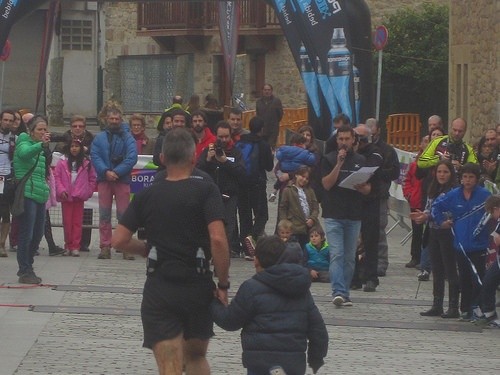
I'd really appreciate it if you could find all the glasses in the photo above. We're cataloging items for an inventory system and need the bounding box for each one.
[71,125,85,129]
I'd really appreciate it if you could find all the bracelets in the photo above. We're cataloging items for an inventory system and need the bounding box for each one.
[218,281,230,289]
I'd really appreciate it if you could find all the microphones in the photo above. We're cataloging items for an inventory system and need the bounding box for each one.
[341,144,347,161]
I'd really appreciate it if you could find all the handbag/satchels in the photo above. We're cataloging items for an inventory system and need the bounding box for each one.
[3,179,28,217]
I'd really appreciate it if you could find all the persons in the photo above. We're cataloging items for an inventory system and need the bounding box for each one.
[409,160,460,318]
[209,235,329,375]
[475,196,500,328]
[319,127,371,306]
[53,139,97,256]
[268,114,400,292]
[256,84,283,150]
[110,128,230,375]
[153,94,274,261]
[0,110,69,257]
[431,162,493,322]
[52,115,94,153]
[13,119,52,284]
[400,115,500,281]
[89,109,138,260]
[129,115,149,154]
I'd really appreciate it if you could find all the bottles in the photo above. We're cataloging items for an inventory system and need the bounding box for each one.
[316,55,325,75]
[299,41,315,73]
[326,26,350,77]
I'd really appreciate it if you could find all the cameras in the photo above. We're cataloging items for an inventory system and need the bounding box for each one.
[213,137,228,154]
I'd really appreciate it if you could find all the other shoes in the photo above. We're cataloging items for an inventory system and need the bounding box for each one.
[16,271,35,277]
[377,268,387,277]
[18,274,41,284]
[362,280,375,292]
[123,252,135,259]
[63,249,71,256]
[475,311,498,327]
[342,297,353,306]
[49,246,67,256]
[441,308,461,318]
[457,311,473,321]
[348,280,362,290]
[71,249,80,257]
[10,248,17,252]
[245,235,256,256]
[98,247,111,259]
[417,270,429,280]
[332,294,344,306]
[405,259,420,267]
[231,250,240,258]
[269,193,276,202]
[419,303,444,316]
[243,251,255,260]
[0,245,7,257]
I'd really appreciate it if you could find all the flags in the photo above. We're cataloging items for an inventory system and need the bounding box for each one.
[267,0,376,132]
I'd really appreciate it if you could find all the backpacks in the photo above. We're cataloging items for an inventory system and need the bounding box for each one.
[223,137,265,178]
